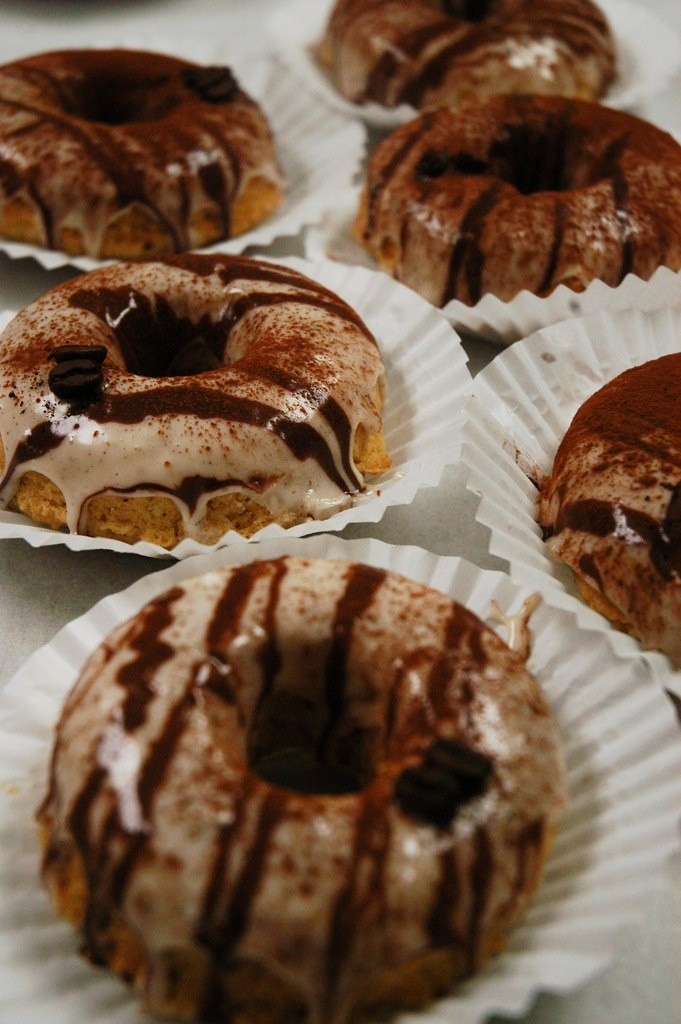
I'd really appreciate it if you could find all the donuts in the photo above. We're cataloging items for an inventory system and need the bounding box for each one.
[1,0,681,1024]
